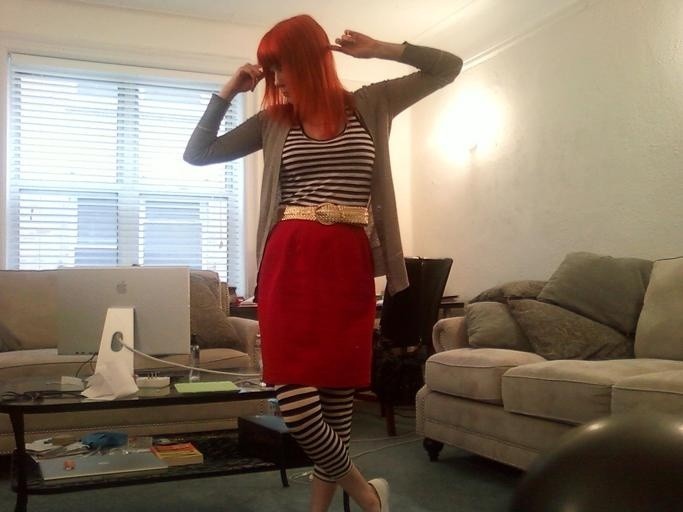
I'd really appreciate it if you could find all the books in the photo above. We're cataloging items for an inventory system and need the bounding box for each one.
[174,381,241,395]
[25,434,91,464]
[240,295,258,308]
[151,442,203,467]
[132,347,252,374]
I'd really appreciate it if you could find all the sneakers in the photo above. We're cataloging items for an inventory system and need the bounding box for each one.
[367,477,390,512]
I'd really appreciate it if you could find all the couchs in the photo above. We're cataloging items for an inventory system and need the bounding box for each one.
[0,264,258,457]
[414,250,680,473]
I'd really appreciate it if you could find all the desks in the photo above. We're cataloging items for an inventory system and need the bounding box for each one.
[226,291,465,403]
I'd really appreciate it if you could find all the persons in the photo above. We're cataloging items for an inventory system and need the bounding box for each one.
[183,15,462,512]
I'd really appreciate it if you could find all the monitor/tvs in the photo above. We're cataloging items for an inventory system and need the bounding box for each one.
[57,267,191,390]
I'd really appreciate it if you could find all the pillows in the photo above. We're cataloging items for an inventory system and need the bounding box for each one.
[461,300,536,355]
[185,267,242,349]
[536,249,652,336]
[509,299,633,361]
[466,277,548,306]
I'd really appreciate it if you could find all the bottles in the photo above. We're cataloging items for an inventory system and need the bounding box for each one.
[187,333,201,381]
[229,286,238,306]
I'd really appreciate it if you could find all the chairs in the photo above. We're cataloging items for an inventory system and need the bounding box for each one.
[353,258,452,432]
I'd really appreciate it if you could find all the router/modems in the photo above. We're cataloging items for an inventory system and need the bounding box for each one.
[136,376,171,388]
